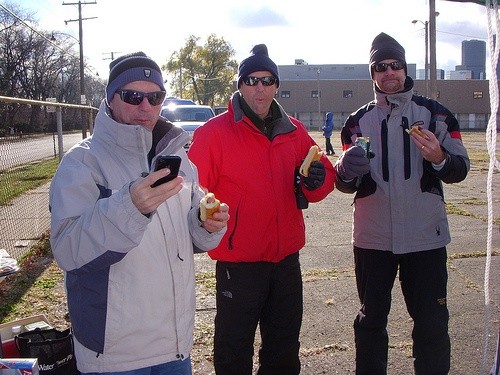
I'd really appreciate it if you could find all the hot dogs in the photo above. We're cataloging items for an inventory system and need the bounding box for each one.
[200,192,220,221]
[405,126,427,139]
[299,145,324,178]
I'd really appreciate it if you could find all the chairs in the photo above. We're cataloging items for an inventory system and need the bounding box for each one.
[166,114,175,121]
[196,113,205,120]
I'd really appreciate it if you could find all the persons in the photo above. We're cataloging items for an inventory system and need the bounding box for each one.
[333,32,470,375]
[188,44,336,375]
[49,52,230,375]
[322,112,335,155]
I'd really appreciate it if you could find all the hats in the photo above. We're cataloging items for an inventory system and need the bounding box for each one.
[238,44,280,87]
[105,51,165,101]
[369,32,407,76]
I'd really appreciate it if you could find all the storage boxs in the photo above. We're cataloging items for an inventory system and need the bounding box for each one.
[0,314,50,360]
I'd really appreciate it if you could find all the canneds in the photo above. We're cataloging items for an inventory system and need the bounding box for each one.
[355,136,371,159]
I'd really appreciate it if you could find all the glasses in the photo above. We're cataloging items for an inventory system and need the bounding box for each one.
[116,88,165,106]
[375,61,405,72]
[243,76,277,86]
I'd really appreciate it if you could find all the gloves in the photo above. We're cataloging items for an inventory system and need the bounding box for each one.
[337,147,375,180]
[301,159,326,189]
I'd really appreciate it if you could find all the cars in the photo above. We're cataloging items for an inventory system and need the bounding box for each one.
[160,105,228,156]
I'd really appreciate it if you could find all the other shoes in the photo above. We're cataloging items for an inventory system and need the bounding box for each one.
[326,151,330,155]
[331,152,335,154]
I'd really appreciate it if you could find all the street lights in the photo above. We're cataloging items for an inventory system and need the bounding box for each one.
[316,68,323,132]
[411,11,441,99]
[50,30,87,140]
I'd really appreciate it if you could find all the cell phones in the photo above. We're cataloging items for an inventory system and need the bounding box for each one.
[153,155,181,187]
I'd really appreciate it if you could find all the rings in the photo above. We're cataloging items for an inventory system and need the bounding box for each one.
[421,146,425,150]
